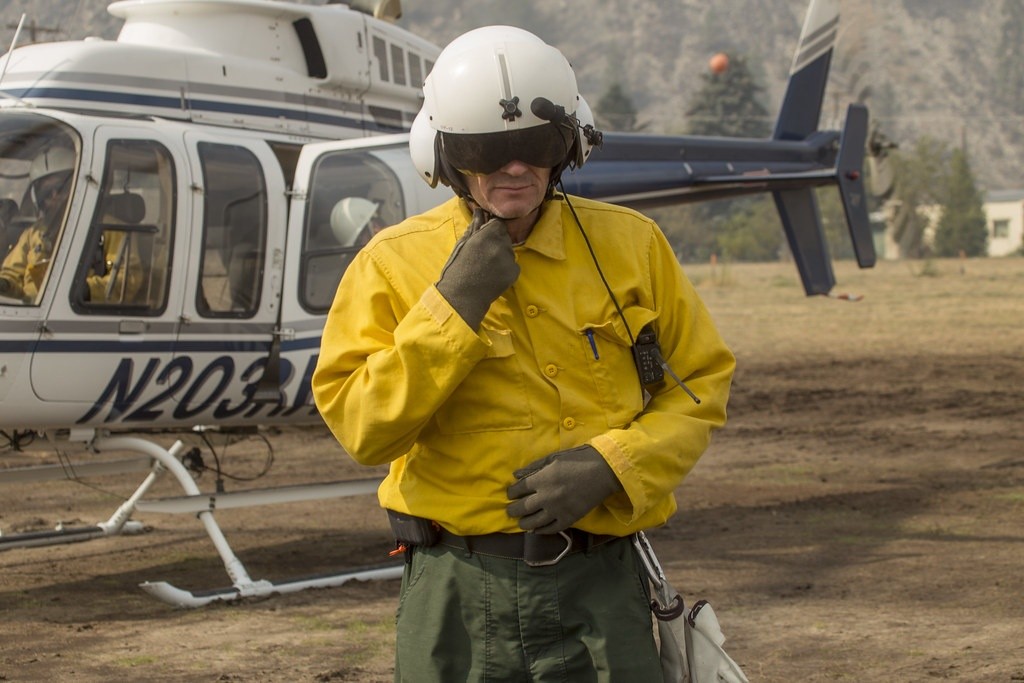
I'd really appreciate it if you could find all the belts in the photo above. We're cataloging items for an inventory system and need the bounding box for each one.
[432,522,616,566]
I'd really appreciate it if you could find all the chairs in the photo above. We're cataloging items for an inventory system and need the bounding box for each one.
[108,191,156,307]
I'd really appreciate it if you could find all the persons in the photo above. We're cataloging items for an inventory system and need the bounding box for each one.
[312,26,737,683]
[0,146,144,302]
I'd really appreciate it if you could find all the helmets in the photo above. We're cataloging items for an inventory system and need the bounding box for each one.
[29,147,76,185]
[329,195,381,257]
[410,24,594,189]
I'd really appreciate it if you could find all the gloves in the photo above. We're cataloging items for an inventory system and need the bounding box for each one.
[433,204,522,333]
[500,444,625,535]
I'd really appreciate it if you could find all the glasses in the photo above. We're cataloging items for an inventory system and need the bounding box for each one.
[438,121,577,174]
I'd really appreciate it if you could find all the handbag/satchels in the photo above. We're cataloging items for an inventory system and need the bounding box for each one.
[648,591,749,683]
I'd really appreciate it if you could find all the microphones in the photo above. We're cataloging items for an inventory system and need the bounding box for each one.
[531,97,604,154]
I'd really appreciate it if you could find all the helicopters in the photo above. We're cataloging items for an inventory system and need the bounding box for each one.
[0,0,876,608]
